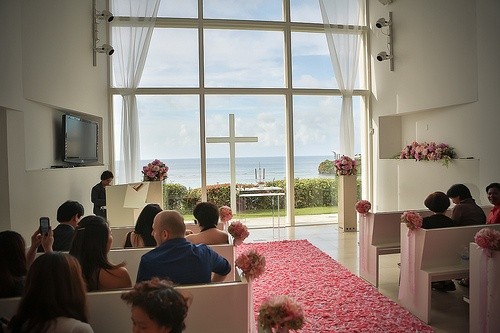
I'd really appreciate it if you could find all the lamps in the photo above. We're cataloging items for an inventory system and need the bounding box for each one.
[377,51,390,62]
[96,9,114,23]
[96,44,115,56]
[376,18,389,29]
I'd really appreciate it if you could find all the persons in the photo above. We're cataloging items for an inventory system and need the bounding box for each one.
[37,200,84,253]
[0,228,54,299]
[458,183,500,288]
[421,192,456,291]
[69,215,132,290]
[121,280,189,333]
[183,202,229,244]
[447,184,486,226]
[136,210,231,284]
[124,204,163,249]
[91,171,114,219]
[7,250,93,333]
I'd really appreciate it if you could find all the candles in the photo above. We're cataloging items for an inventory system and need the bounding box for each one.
[263,168,265,179]
[255,168,257,179]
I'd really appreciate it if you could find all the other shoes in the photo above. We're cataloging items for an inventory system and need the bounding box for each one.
[434,282,456,291]
[458,278,469,287]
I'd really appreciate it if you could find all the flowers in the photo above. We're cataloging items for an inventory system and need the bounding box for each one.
[354,199,372,215]
[400,140,457,169]
[233,247,267,280]
[257,293,304,333]
[333,154,358,176]
[473,227,500,257]
[399,211,426,232]
[228,220,251,245]
[141,159,170,182]
[218,205,232,222]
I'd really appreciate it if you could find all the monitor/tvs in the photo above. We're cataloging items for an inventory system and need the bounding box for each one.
[60,115,99,163]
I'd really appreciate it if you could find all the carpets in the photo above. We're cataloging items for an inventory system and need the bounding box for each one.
[227,239,432,333]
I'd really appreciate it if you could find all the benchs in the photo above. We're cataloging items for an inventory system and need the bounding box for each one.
[356,211,500,333]
[0,221,252,333]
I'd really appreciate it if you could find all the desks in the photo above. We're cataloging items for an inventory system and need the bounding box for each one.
[237,188,286,219]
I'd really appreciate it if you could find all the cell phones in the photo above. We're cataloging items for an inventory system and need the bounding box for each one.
[40,217,50,236]
